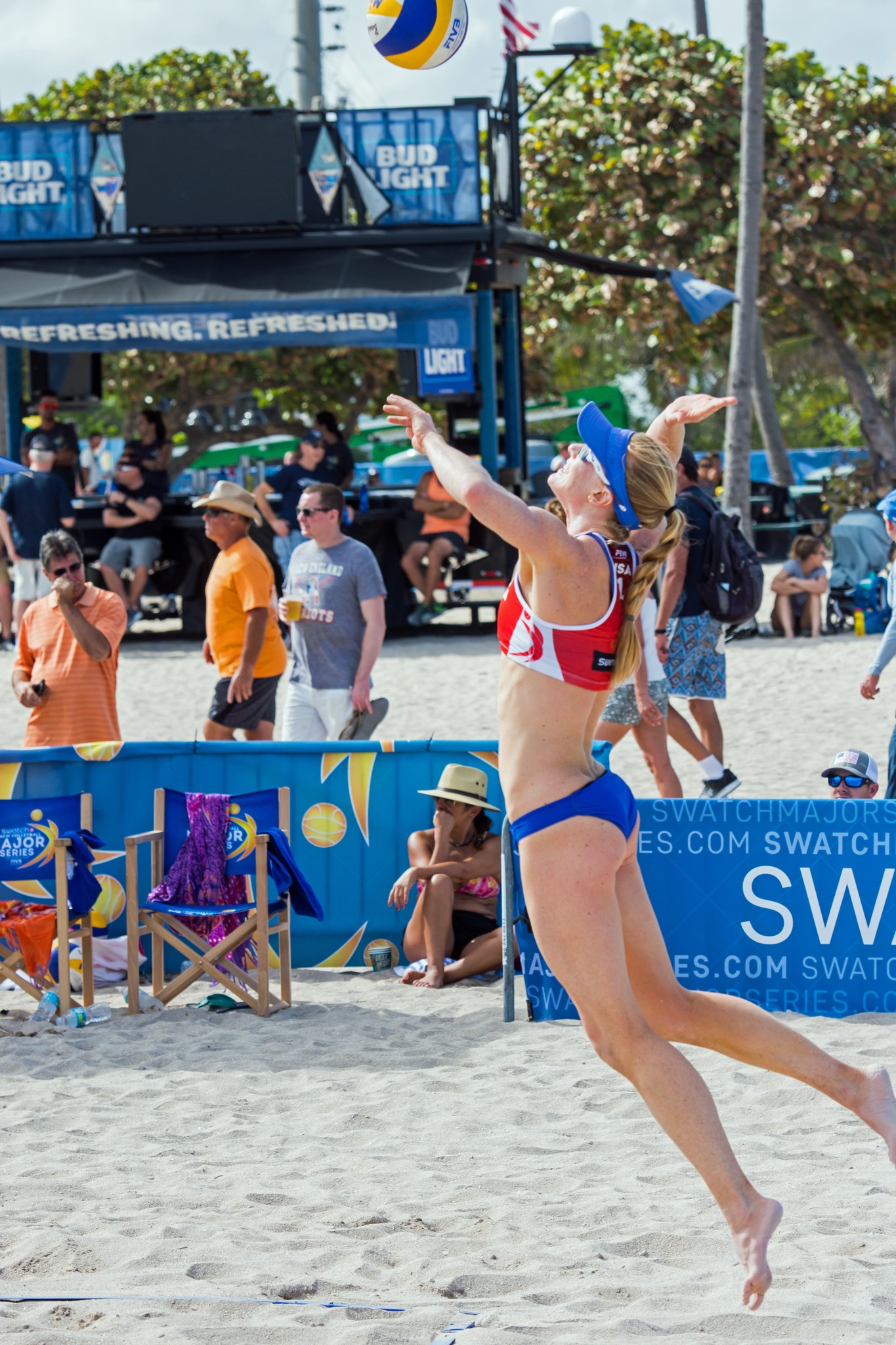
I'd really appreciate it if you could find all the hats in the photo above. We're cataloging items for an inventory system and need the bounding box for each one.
[118,453,142,466]
[417,763,502,813]
[679,446,699,471]
[822,749,878,784]
[316,411,345,440]
[302,429,330,445]
[191,480,264,528]
[577,400,644,531]
[30,434,57,452]
[876,488,896,522]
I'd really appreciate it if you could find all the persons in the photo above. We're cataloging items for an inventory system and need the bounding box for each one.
[861,489,896,799]
[0,536,13,645]
[19,387,77,500]
[98,461,162,630]
[278,483,387,742]
[80,428,112,496]
[770,535,830,638]
[0,435,76,645]
[387,763,519,990]
[821,748,878,799]
[382,393,896,1312]
[592,516,687,798]
[252,431,354,582]
[192,481,287,742]
[116,407,173,496]
[314,409,355,491]
[401,470,470,626]
[653,445,742,799]
[11,529,128,749]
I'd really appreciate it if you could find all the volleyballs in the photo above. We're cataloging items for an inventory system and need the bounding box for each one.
[42,941,83,993]
[365,0,468,70]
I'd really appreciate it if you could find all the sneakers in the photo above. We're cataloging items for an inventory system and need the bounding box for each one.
[699,768,742,799]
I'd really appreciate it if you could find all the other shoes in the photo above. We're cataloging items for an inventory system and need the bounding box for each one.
[126,608,143,629]
[0,633,15,651]
[408,602,445,625]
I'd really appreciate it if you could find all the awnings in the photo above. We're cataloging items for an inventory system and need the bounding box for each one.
[0,457,34,476]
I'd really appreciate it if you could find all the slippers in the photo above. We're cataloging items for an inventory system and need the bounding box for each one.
[186,993,252,1013]
[339,698,388,740]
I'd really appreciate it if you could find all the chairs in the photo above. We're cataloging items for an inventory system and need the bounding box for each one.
[125,787,291,1017]
[0,792,94,1015]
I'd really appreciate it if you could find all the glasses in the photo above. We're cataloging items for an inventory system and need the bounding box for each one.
[432,795,458,807]
[701,464,712,468]
[51,560,82,577]
[205,506,234,519]
[41,402,60,408]
[828,774,872,788]
[311,442,327,450]
[816,550,825,557]
[115,465,132,472]
[579,443,627,513]
[295,506,332,517]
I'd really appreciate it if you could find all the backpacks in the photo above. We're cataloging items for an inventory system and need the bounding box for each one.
[677,492,764,625]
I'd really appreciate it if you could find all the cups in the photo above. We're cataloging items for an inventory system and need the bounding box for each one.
[282,587,303,621]
[368,946,392,972]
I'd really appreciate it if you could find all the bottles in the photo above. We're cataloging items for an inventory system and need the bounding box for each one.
[181,961,216,978]
[853,608,865,636]
[29,983,60,1021]
[55,1001,112,1028]
[121,987,164,1013]
